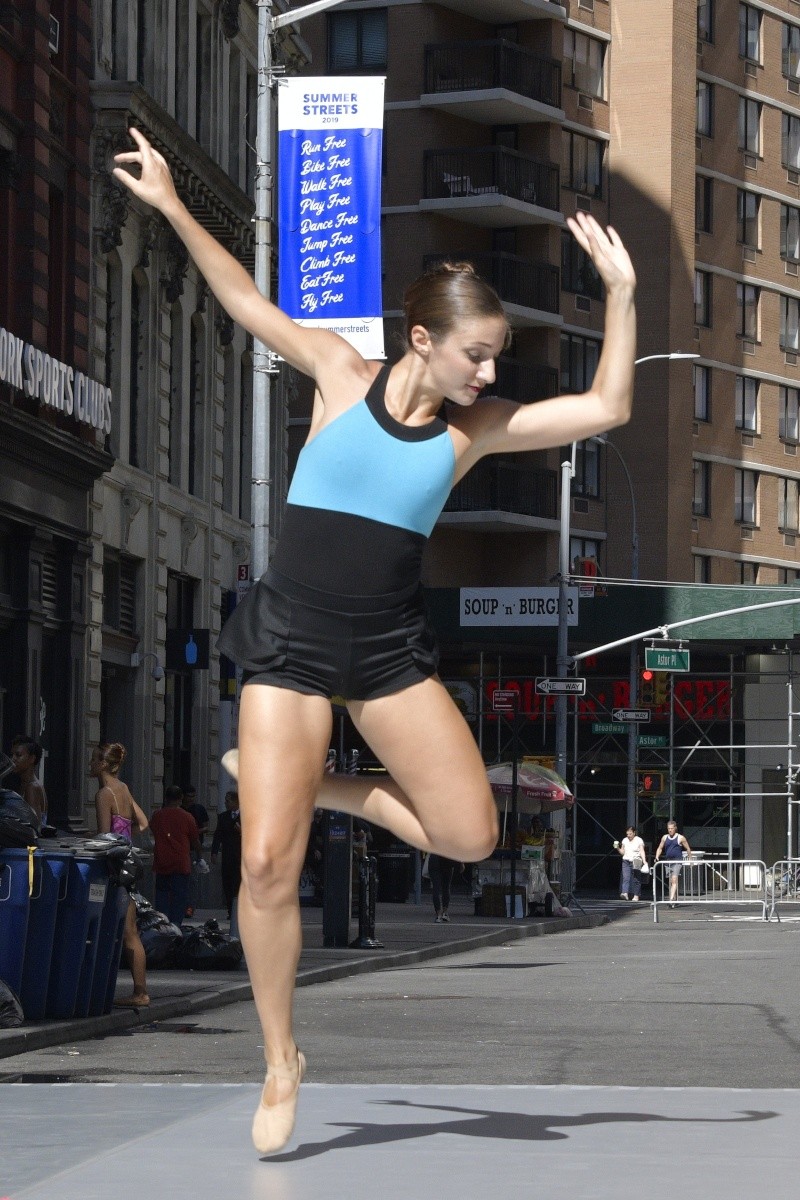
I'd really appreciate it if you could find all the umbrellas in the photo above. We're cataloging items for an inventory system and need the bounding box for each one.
[485,760,575,851]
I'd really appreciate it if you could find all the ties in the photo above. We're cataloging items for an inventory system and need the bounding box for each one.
[233,810,238,822]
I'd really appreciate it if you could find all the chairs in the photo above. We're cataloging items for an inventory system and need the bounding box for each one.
[442,172,500,198]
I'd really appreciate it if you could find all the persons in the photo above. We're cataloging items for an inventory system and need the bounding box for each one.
[421,851,465,923]
[612,826,649,902]
[654,821,691,910]
[0,732,243,1007]
[111,126,637,1153]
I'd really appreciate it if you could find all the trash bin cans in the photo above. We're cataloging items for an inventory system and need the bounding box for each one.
[0,835,155,1021]
[377,853,411,902]
[679,851,706,895]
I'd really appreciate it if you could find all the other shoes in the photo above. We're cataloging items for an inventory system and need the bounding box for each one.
[184,905,193,918]
[435,915,442,922]
[631,895,639,901]
[620,892,628,900]
[666,900,679,909]
[441,912,450,921]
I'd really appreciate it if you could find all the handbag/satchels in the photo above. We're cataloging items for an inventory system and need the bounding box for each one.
[421,852,432,880]
[633,855,643,870]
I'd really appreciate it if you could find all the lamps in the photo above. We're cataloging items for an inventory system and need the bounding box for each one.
[141,653,165,680]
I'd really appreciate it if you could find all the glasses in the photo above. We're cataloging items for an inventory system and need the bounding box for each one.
[226,800,232,803]
